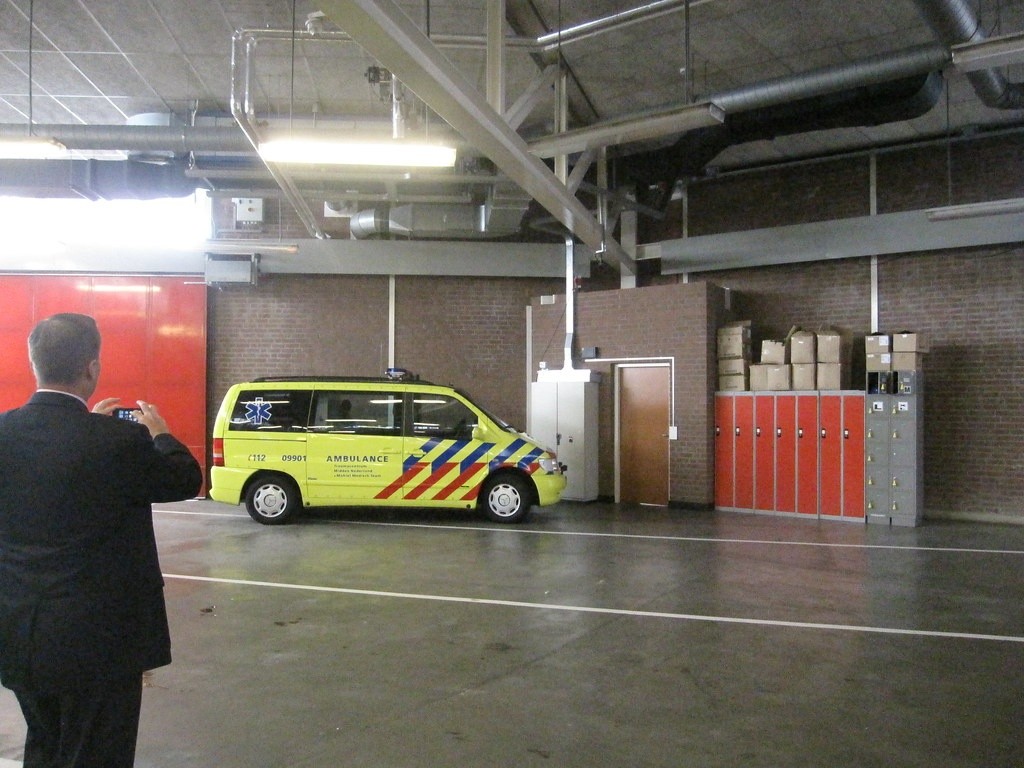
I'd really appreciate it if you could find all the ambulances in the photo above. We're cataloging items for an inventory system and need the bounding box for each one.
[207,367,568,527]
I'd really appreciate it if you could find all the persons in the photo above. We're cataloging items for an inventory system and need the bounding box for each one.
[0,312,202,768]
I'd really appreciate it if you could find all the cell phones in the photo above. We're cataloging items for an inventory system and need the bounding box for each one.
[112,407,143,423]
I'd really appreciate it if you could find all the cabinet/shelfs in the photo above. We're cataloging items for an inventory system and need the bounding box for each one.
[865,369,924,527]
[530,382,598,503]
[713,391,865,523]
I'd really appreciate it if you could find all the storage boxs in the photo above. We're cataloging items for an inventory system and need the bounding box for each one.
[891,352,923,369]
[865,334,891,353]
[866,354,891,371]
[717,321,853,391]
[894,333,930,352]
